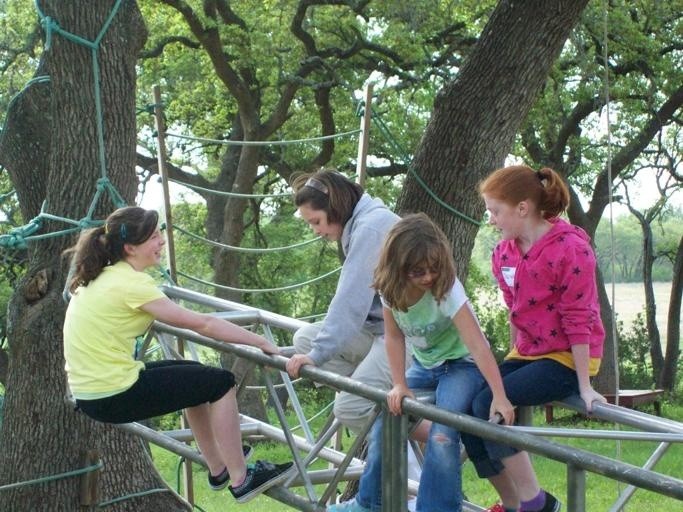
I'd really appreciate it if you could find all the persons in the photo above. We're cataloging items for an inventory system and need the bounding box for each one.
[456,164,611,512]
[279,164,469,466]
[62,205,300,504]
[327,211,519,512]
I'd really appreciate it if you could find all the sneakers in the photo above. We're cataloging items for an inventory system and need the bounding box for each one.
[324,498,370,512]
[206,444,251,490]
[226,461,295,502]
[479,490,562,511]
